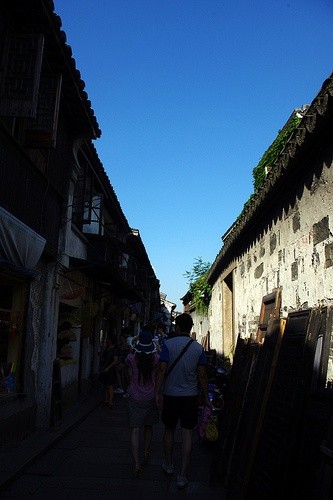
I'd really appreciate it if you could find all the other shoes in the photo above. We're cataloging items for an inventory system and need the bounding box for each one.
[99,401,112,410]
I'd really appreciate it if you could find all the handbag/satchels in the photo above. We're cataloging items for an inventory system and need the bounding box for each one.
[198,407,218,443]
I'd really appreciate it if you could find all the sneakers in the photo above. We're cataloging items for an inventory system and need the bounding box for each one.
[175,474,188,489]
[159,462,174,476]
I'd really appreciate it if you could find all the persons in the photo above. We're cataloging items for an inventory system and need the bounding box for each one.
[113,319,176,398]
[156,313,212,491]
[99,334,121,410]
[125,327,161,480]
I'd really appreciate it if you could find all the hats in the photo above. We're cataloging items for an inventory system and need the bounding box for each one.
[126,330,155,354]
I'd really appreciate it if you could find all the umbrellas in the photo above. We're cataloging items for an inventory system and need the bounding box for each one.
[60,278,84,300]
